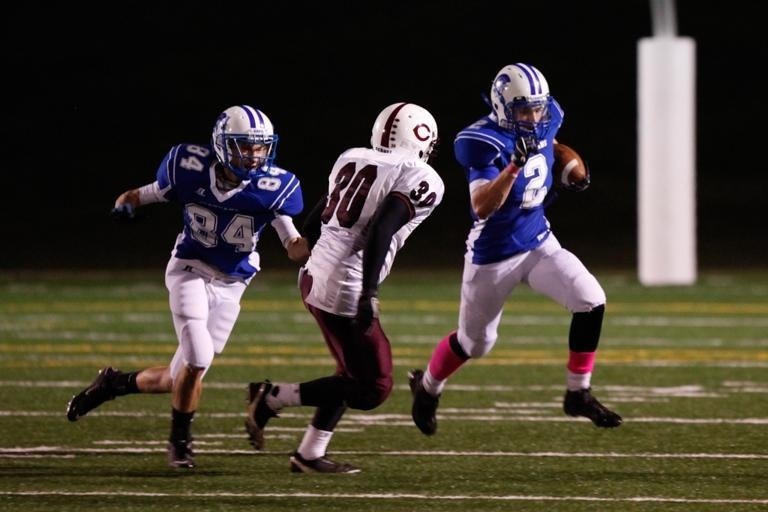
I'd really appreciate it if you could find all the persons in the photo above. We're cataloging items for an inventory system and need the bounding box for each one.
[408,62,624,435]
[245,100,445,476]
[64,104,310,470]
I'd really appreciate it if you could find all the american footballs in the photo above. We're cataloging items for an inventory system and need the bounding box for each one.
[554,144,585,186]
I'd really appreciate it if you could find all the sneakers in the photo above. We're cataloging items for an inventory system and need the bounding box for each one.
[409,369,441,434]
[67,367,122,422]
[564,388,622,427]
[288,453,362,474]
[169,435,196,472]
[245,380,281,450]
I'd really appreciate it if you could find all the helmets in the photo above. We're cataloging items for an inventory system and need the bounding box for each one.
[370,103,440,165]
[490,62,553,140]
[212,105,279,178]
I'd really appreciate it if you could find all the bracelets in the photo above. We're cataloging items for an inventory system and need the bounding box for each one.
[505,163,522,178]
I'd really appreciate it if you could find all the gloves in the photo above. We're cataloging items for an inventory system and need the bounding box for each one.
[353,296,378,336]
[512,137,536,167]
